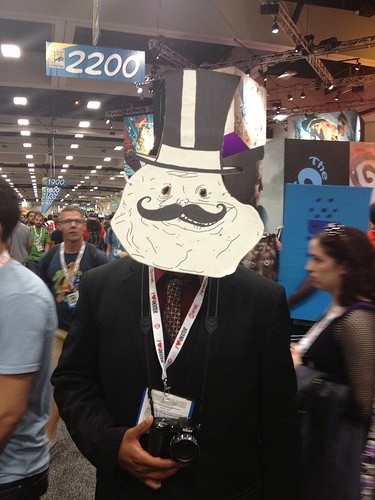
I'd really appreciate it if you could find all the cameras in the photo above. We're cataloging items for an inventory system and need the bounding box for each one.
[148,417,206,463]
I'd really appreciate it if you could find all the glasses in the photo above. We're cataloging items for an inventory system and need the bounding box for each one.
[321,222,363,266]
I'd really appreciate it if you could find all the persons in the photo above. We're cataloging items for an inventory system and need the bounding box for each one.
[230,146,274,236]
[290,222,375,500]
[39,205,112,450]
[6,210,129,275]
[0,175,58,500]
[50,78,299,500]
